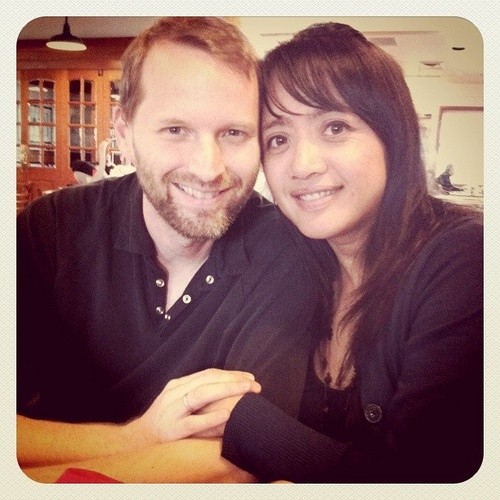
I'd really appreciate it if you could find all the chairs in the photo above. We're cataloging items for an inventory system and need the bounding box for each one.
[16,179,37,214]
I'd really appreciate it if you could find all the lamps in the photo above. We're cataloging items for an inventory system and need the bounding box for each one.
[46,17,87,50]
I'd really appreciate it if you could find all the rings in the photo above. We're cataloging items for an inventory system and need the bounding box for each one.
[182,393,194,414]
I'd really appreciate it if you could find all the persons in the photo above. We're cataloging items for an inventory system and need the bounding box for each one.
[190,22,484,484]
[436,164,467,191]
[15,16,322,484]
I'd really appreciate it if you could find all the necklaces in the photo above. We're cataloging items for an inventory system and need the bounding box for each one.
[318,280,359,416]
